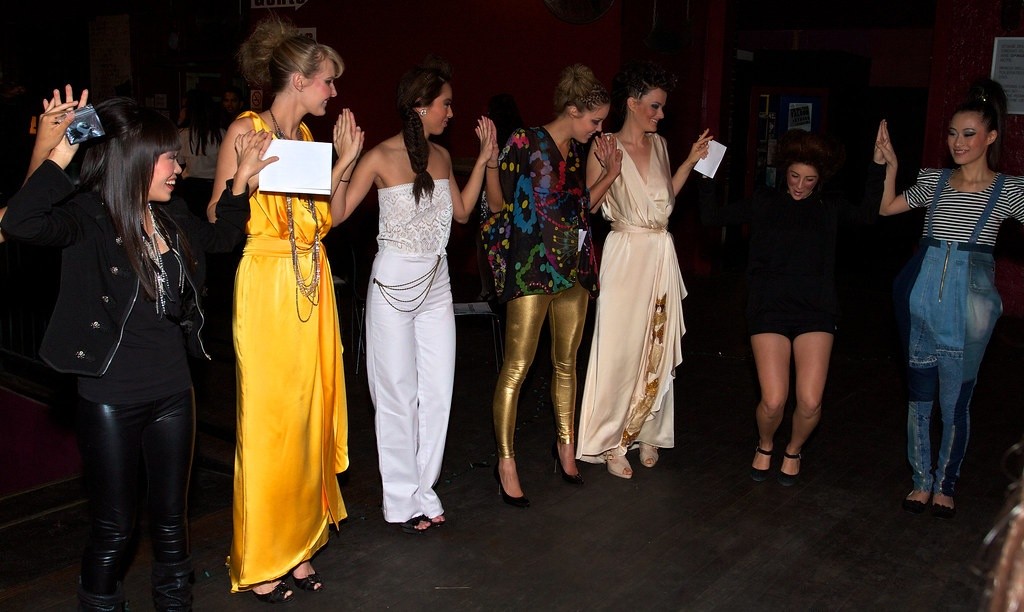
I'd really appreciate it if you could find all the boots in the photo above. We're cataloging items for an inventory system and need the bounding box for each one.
[78,582,124,612]
[151,554,192,612]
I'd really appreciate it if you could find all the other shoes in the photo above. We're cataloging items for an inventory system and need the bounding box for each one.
[903,489,931,514]
[933,492,956,519]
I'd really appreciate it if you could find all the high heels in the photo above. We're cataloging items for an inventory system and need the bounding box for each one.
[750,443,773,481]
[605,447,633,478]
[250,580,294,604]
[494,457,530,507]
[423,515,446,527]
[398,516,432,534]
[291,561,323,591]
[777,444,802,486]
[552,439,584,484]
[639,441,658,467]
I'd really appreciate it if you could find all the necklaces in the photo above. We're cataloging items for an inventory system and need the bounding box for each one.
[265,105,323,324]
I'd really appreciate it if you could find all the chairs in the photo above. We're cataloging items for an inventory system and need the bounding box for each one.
[350,241,366,375]
[454,303,504,373]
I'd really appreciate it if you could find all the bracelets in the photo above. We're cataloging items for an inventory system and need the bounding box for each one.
[485,164,498,169]
[340,179,349,183]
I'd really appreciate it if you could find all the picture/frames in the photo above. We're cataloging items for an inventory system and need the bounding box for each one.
[990,36,1024,115]
[776,96,820,144]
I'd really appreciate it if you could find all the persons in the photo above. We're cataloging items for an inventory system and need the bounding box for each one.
[875,78,1024,520]
[204,22,366,598]
[584,68,715,478]
[729,150,861,488]
[471,62,623,507]
[330,62,493,532]
[0,85,246,612]
